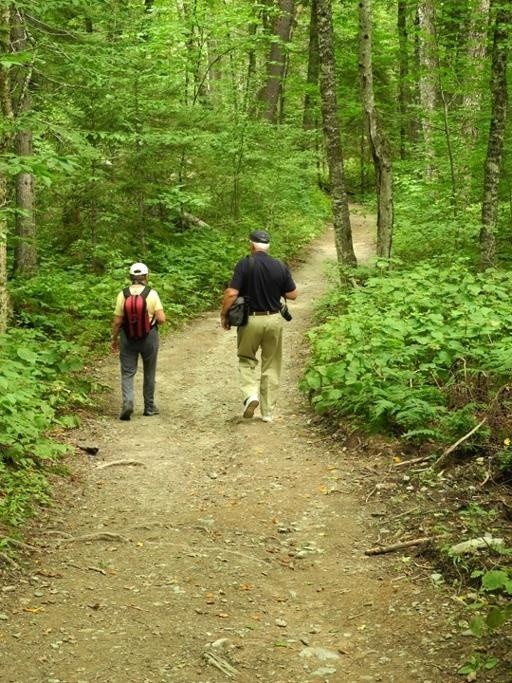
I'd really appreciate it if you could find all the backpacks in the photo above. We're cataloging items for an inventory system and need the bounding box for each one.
[123,286,155,340]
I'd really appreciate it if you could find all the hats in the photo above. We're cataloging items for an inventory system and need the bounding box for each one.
[129,263,148,275]
[249,231,268,243]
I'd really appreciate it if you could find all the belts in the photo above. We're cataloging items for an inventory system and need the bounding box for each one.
[249,311,279,315]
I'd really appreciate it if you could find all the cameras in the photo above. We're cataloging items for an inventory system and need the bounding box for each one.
[279,301,293,321]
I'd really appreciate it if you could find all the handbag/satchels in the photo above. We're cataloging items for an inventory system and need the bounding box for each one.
[227,297,248,326]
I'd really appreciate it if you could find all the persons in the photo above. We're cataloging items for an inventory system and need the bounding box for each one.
[109,260,168,420]
[219,229,301,422]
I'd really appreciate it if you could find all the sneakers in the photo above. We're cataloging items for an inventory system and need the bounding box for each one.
[120,405,133,420]
[243,396,273,421]
[143,407,160,415]
